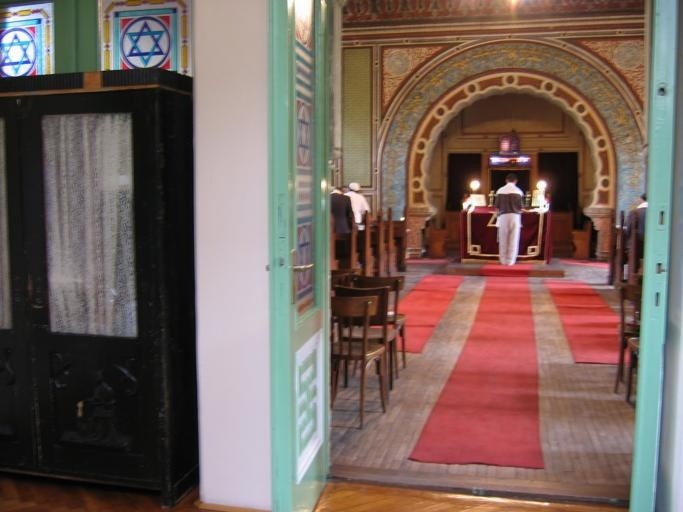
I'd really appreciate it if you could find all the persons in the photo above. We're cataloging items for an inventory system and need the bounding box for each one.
[344,181,370,224]
[494,173,524,266]
[627,193,647,259]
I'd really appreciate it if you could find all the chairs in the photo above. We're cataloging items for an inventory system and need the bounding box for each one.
[330,267,406,429]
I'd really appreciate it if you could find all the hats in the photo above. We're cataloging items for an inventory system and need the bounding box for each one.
[349,183,361,191]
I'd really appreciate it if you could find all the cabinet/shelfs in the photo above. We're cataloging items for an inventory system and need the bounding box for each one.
[0,65,200,510]
